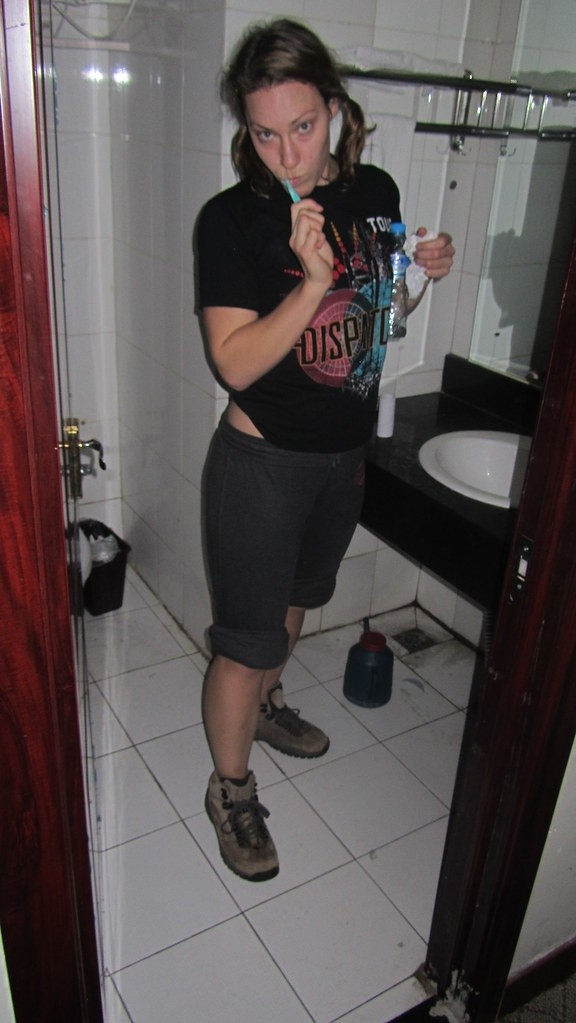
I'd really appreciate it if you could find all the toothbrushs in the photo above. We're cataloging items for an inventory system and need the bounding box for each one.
[281,172,309,217]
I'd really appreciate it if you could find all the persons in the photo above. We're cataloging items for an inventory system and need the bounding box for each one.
[192,15,455,881]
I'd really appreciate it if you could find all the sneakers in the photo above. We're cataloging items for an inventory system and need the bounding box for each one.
[204,765,279,882]
[259,685,330,759]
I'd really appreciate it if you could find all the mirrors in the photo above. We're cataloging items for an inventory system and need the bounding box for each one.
[469,2,575,367]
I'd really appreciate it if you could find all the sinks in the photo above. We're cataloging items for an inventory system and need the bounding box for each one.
[417,429,554,511]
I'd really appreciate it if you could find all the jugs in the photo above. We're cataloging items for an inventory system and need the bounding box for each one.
[341,612,394,707]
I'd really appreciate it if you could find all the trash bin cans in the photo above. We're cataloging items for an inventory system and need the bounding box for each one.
[78,517,132,617]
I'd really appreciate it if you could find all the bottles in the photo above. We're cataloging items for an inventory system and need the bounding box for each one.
[386,221,411,338]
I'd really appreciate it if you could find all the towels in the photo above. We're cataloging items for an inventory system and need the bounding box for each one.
[401,230,440,301]
[334,41,467,79]
